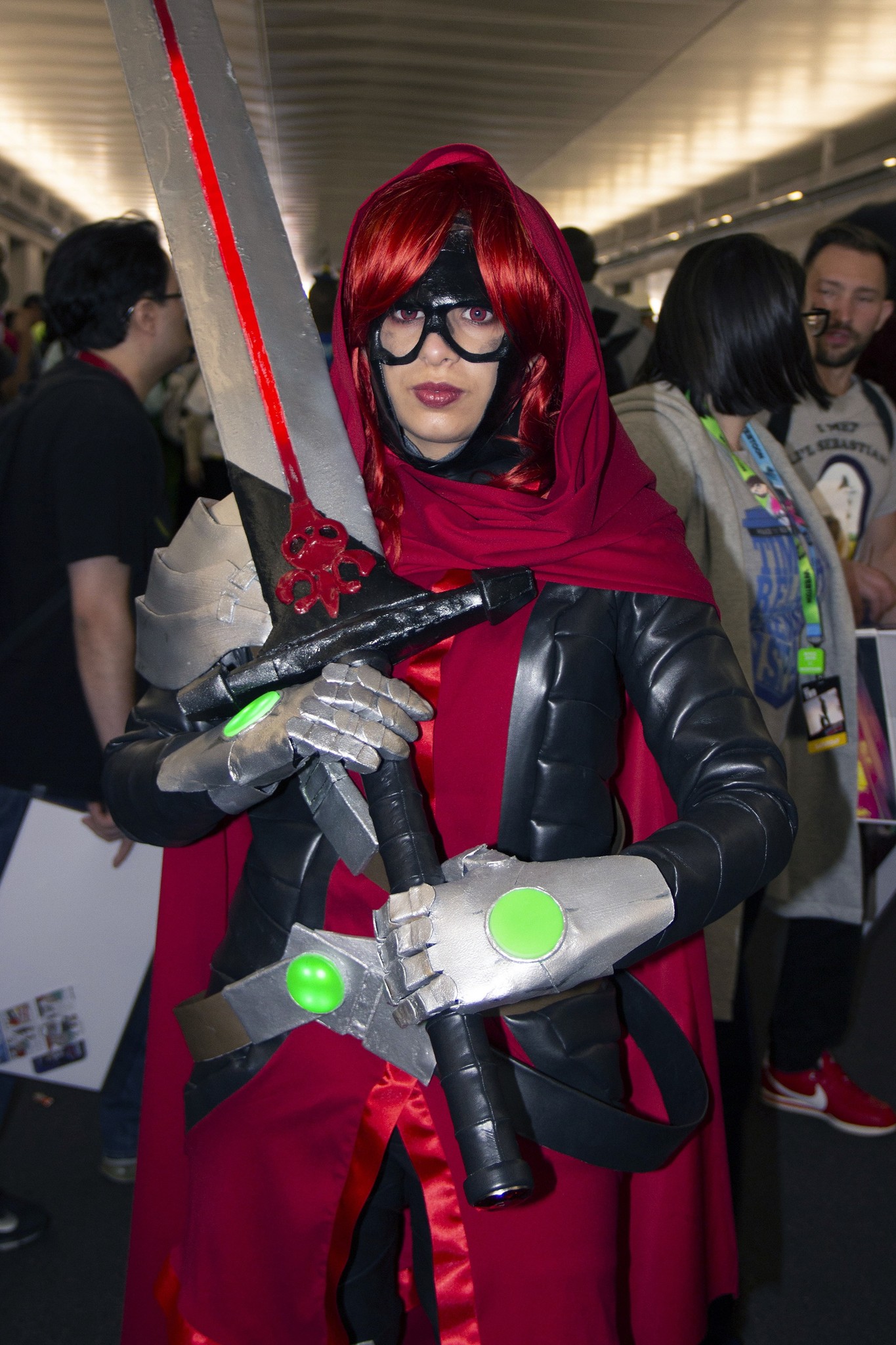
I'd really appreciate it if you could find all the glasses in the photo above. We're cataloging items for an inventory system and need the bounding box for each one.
[802,308,830,337]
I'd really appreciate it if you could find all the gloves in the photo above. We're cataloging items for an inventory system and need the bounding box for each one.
[156,663,432,815]
[372,843,674,1029]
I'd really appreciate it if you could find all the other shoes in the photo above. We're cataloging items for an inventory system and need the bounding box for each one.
[102,1156,136,1184]
[0,1200,47,1252]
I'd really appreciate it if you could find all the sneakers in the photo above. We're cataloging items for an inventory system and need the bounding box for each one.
[762,1050,896,1137]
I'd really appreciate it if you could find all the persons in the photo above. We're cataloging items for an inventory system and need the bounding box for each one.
[0,142,896,1345]
